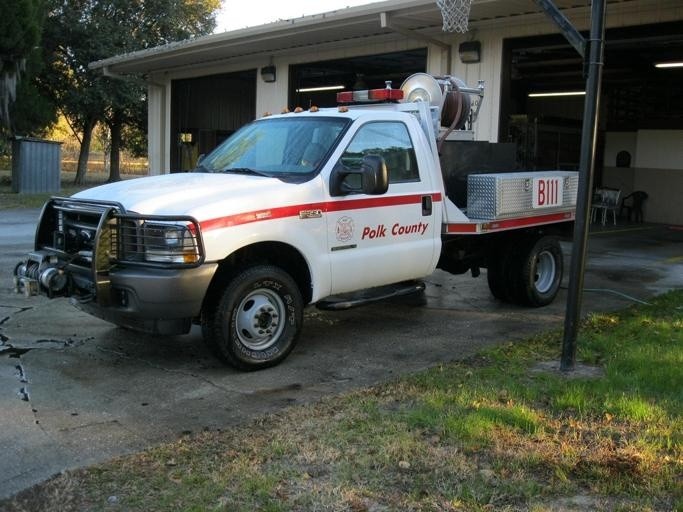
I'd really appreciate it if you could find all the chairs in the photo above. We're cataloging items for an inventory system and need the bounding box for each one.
[295,142,406,180]
[589,188,649,225]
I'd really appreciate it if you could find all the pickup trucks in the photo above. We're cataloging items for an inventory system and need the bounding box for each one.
[27,84,580,372]
